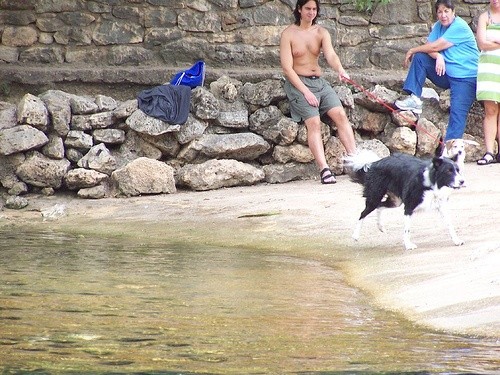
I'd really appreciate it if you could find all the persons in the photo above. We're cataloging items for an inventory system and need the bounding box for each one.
[280,0,356,184]
[395,0,480,139]
[476,0,500,165]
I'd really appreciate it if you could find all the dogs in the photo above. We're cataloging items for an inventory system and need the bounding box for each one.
[339,138,470,250]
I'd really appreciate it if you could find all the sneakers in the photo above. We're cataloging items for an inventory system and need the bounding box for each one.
[395,93,423,113]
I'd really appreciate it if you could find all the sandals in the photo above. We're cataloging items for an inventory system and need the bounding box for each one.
[477,152,497,164]
[320,168,336,184]
